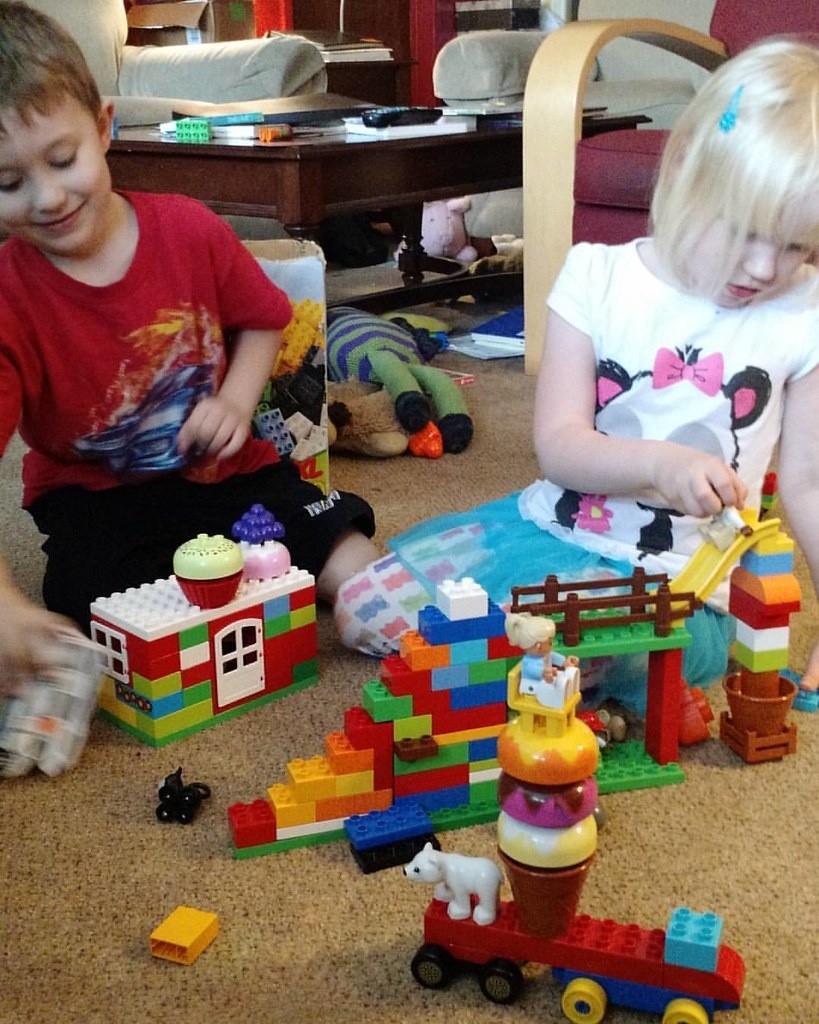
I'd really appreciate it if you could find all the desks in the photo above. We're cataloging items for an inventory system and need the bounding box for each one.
[105,113,654,314]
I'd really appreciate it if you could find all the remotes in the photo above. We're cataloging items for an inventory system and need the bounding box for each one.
[363,107,443,129]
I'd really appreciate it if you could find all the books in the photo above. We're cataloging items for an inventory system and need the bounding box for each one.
[470,301,523,351]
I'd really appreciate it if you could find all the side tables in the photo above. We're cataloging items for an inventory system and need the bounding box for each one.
[326,55,421,107]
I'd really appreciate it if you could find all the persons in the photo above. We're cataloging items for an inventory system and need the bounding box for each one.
[335,34,819,693]
[504,610,582,708]
[0,0,381,701]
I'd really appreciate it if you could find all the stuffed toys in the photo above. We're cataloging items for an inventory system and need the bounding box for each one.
[269,302,478,458]
[394,195,522,260]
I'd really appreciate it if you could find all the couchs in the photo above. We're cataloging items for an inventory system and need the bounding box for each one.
[23,0,328,240]
[433,0,717,238]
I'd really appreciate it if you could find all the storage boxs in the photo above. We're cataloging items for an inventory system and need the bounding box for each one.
[123,0,257,46]
[226,237,331,496]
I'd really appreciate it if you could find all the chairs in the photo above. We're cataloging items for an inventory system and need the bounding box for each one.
[523,0,819,378]
